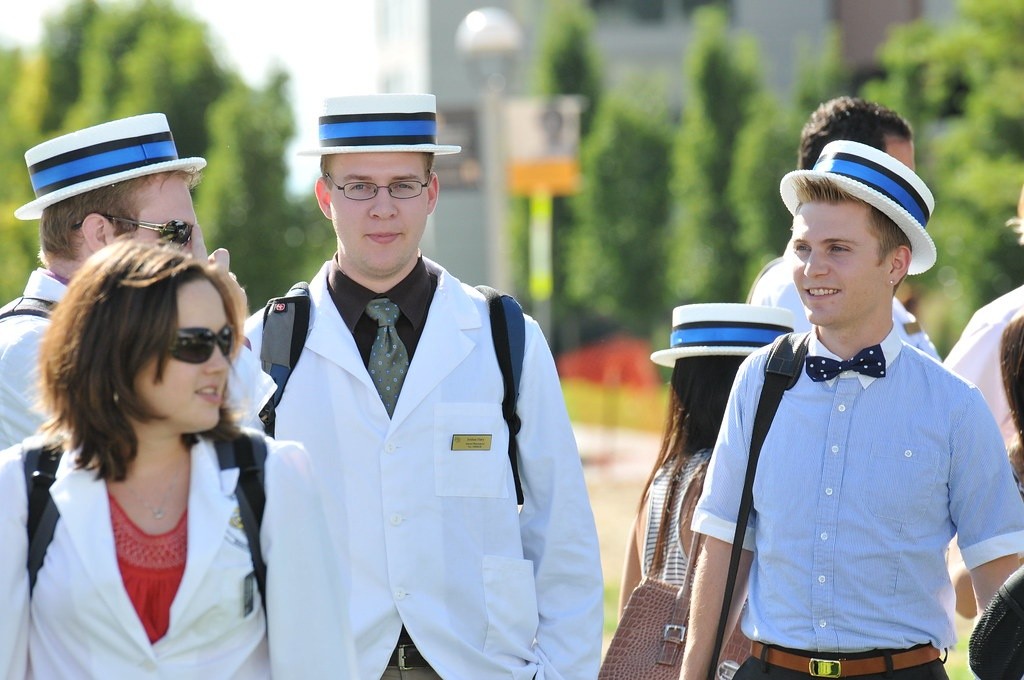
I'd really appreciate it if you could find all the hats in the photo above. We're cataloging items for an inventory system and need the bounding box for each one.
[297,93,461,156]
[651,301,796,368]
[14,111,207,221]
[780,141,938,275]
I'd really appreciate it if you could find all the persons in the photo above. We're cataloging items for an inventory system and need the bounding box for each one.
[0,113,278,453]
[244,94,604,680]
[617,303,794,680]
[679,141,1024,680]
[0,240,359,680]
[746,96,943,363]
[943,184,1024,621]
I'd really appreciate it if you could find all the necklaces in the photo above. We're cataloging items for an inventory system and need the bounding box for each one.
[145,475,177,519]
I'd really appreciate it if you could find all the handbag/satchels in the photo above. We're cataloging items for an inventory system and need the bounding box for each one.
[594,578,753,680]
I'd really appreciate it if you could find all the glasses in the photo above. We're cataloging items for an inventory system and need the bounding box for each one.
[325,171,430,200]
[165,325,234,363]
[69,213,195,248]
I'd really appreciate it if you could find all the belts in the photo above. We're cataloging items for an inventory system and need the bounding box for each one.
[750,634,946,678]
[388,647,428,671]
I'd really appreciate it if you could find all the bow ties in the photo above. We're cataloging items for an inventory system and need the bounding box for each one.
[805,344,888,383]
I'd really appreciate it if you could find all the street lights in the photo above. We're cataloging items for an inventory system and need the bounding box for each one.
[460,8,528,291]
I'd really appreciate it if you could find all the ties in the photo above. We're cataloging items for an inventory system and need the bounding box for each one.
[363,299,409,420]
